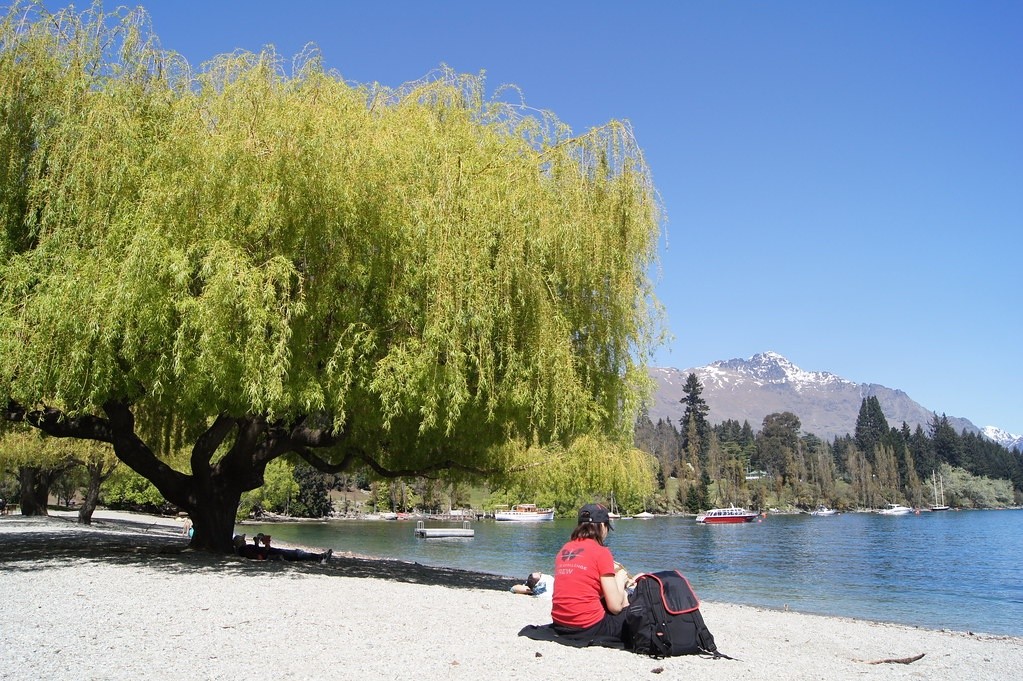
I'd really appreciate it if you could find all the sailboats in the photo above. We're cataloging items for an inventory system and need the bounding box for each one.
[928,470,951,511]
[632,498,655,518]
[607,490,621,518]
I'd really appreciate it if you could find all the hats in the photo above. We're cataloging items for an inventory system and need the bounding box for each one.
[578,503,613,531]
[234,534,245,545]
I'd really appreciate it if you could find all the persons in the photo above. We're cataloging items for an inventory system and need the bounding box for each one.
[181,519,194,537]
[551,502,634,645]
[234,533,332,565]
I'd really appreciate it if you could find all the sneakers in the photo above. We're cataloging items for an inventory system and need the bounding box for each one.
[322,549,332,563]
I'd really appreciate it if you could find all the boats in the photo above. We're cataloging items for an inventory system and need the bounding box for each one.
[695,502,761,525]
[494,503,555,521]
[810,505,835,516]
[620,510,633,520]
[414,521,475,538]
[877,503,911,516]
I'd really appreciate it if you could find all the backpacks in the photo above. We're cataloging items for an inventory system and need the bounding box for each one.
[626,570,736,659]
